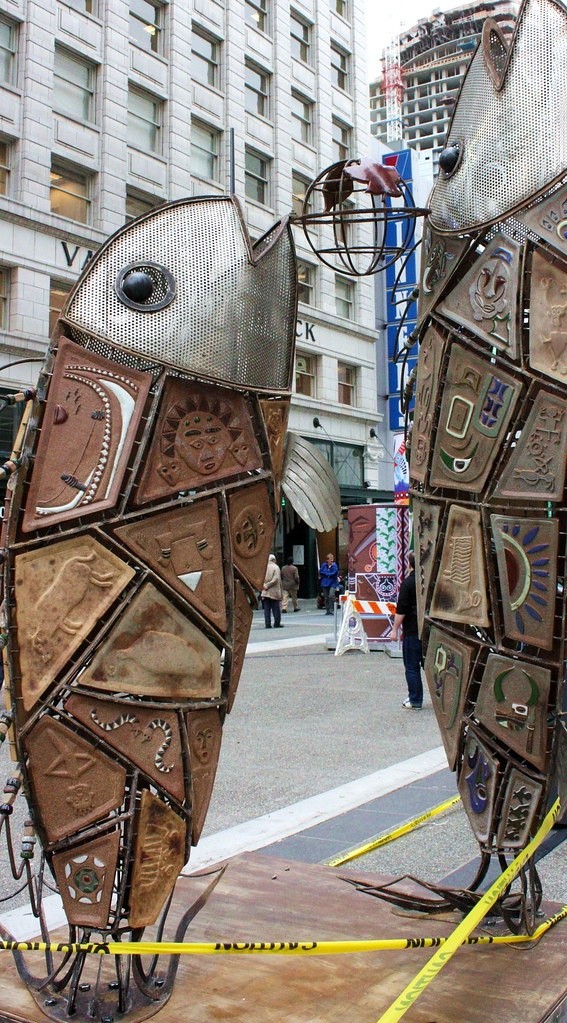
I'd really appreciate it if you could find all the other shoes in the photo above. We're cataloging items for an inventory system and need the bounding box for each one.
[294,608,301,613]
[325,612,334,615]
[265,624,272,627]
[274,624,283,627]
[282,608,287,612]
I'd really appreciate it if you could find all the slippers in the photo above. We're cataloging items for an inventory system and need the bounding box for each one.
[404,699,422,711]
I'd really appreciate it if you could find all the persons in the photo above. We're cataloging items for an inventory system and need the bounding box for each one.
[279,556,302,613]
[258,553,283,629]
[319,553,340,615]
[389,553,429,710]
